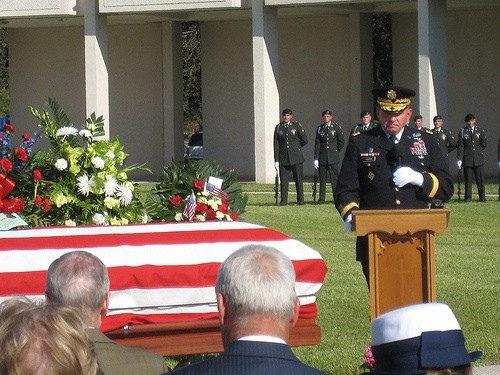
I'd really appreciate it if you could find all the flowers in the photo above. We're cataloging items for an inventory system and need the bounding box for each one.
[169,181,238,224]
[359,346,377,373]
[0,94,155,228]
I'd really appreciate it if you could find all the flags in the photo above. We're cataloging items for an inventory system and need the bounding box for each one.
[206,183,227,200]
[183,193,197,221]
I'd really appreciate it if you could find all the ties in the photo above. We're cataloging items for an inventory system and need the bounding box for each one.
[436,130,439,135]
[470,128,474,135]
[325,125,330,131]
[364,126,367,131]
[389,134,397,145]
[284,124,289,130]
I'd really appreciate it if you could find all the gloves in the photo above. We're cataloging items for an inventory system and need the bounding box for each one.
[392,166,424,189]
[274,162,280,172]
[344,215,357,239]
[457,160,462,169]
[313,160,319,170]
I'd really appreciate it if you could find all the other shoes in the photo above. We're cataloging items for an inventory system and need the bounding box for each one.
[479,198,486,203]
[280,200,287,205]
[297,201,304,205]
[464,198,472,203]
[318,199,324,204]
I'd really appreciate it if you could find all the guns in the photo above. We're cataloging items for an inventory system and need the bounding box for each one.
[274,171,278,205]
[312,168,317,203]
[457,162,462,201]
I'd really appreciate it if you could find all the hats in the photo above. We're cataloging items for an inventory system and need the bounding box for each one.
[356,302,483,374]
[370,86,416,115]
[360,111,371,117]
[282,109,292,115]
[322,110,332,116]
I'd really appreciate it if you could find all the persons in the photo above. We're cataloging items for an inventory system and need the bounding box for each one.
[457,113,489,202]
[45,251,169,375]
[371,302,483,375]
[165,245,327,375]
[0,298,104,375]
[274,109,308,204]
[314,110,344,203]
[413,114,430,133]
[334,86,454,291]
[351,111,379,134]
[431,116,457,158]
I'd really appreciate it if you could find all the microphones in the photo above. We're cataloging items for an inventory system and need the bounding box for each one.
[393,142,405,170]
